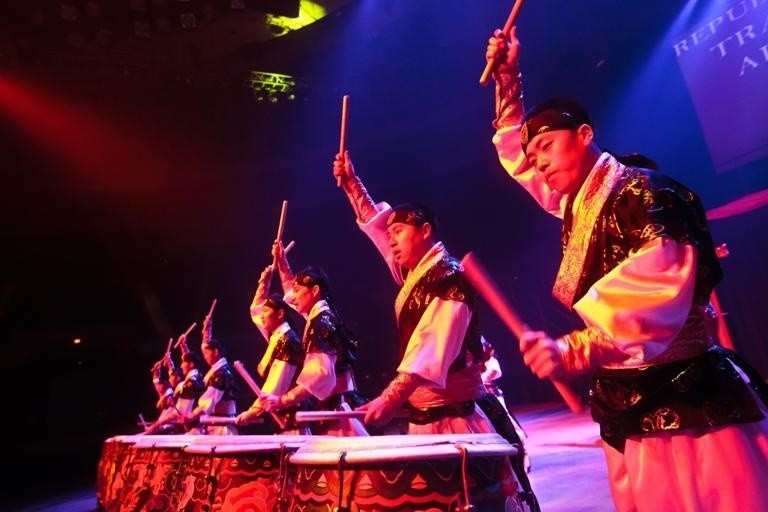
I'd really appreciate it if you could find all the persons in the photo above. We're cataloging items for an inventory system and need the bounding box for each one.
[332,150,541,511]
[234,263,311,435]
[130,316,243,439]
[483,23,768,511]
[260,238,371,436]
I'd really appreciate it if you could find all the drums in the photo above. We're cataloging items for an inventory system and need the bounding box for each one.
[120,435,260,512]
[290,431,521,512]
[97,434,216,512]
[183,433,353,511]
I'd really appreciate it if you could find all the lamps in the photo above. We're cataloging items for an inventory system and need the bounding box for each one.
[244,0,301,18]
